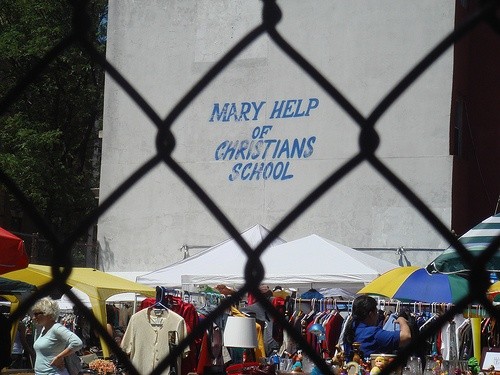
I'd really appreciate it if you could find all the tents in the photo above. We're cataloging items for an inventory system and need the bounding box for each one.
[0,214,401,359]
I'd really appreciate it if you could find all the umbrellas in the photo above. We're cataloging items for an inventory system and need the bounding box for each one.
[357,212,500,305]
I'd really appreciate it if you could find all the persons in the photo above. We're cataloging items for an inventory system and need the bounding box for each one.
[29,297,82,375]
[341,297,411,366]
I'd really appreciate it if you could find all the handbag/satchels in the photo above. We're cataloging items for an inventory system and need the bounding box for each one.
[64,353,81,375]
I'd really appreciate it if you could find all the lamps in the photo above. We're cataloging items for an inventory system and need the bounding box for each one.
[223,316,258,362]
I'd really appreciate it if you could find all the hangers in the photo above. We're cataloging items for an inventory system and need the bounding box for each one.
[149,286,225,315]
[293,297,486,331]
